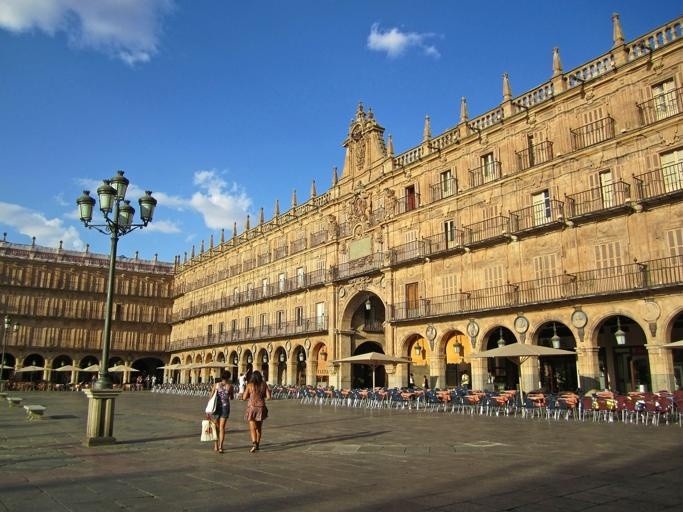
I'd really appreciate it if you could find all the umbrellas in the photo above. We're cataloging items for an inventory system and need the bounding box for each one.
[457,339,581,411]
[155,360,235,386]
[643,339,683,352]
[328,352,416,395]
[0,362,140,391]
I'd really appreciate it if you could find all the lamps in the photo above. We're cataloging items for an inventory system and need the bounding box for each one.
[496,327,505,347]
[233,347,305,363]
[451,333,462,354]
[365,292,371,310]
[551,322,560,348]
[613,316,627,346]
[413,334,421,356]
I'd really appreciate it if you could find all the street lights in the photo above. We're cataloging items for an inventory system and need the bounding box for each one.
[75,169,158,447]
[0,315,18,392]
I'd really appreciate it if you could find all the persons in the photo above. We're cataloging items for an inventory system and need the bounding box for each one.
[238,367,251,393]
[242,371,271,453]
[211,370,234,453]
[423,374,429,389]
[460,370,470,390]
[139,374,156,390]
[409,371,414,388]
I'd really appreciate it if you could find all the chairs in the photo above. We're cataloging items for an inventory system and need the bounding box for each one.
[149,381,297,401]
[297,384,682,428]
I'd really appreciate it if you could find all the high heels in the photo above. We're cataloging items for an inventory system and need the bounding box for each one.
[248,441,259,453]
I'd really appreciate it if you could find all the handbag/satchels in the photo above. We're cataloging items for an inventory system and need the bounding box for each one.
[205,390,218,414]
[201,420,218,442]
